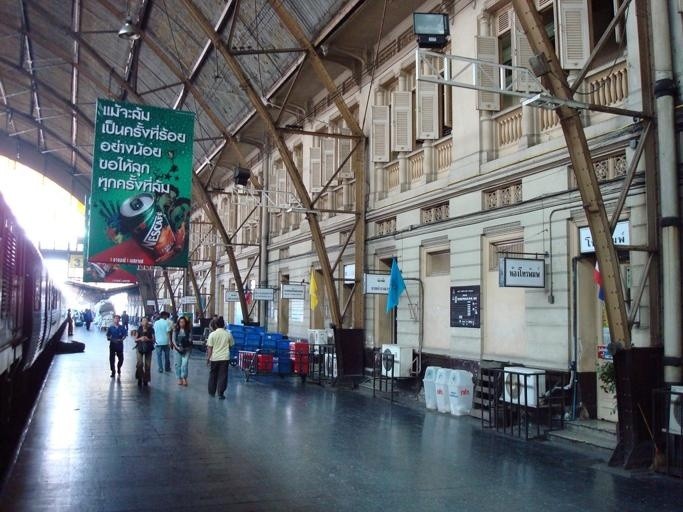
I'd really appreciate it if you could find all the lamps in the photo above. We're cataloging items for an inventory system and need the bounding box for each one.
[117,1,142,38]
[232,168,252,188]
[414,12,453,48]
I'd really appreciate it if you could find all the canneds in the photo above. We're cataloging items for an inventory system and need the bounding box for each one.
[120,193,176,260]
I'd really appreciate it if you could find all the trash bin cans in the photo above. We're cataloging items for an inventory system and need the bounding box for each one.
[422,366,475,416]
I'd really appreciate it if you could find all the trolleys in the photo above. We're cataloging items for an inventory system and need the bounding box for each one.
[230,349,305,383]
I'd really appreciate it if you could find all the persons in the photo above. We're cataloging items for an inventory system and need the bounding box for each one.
[134,312,193,385]
[210,315,218,331]
[66,308,94,336]
[120,311,129,335]
[106,315,126,376]
[206,316,233,399]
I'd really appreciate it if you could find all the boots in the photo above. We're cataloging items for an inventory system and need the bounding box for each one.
[177,378,188,385]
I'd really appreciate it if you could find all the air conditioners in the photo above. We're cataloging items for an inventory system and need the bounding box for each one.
[502,365,548,411]
[378,343,413,380]
[308,328,339,378]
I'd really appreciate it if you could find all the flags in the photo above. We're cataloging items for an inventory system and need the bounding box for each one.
[386,257,405,313]
[309,272,319,311]
[244,284,252,304]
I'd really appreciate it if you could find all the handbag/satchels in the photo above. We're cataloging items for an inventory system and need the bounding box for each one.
[142,342,154,353]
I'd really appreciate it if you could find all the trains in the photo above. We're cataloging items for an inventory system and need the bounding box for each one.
[0,192,67,422]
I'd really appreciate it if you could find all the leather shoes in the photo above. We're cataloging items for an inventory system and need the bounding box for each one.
[211,393,225,399]
